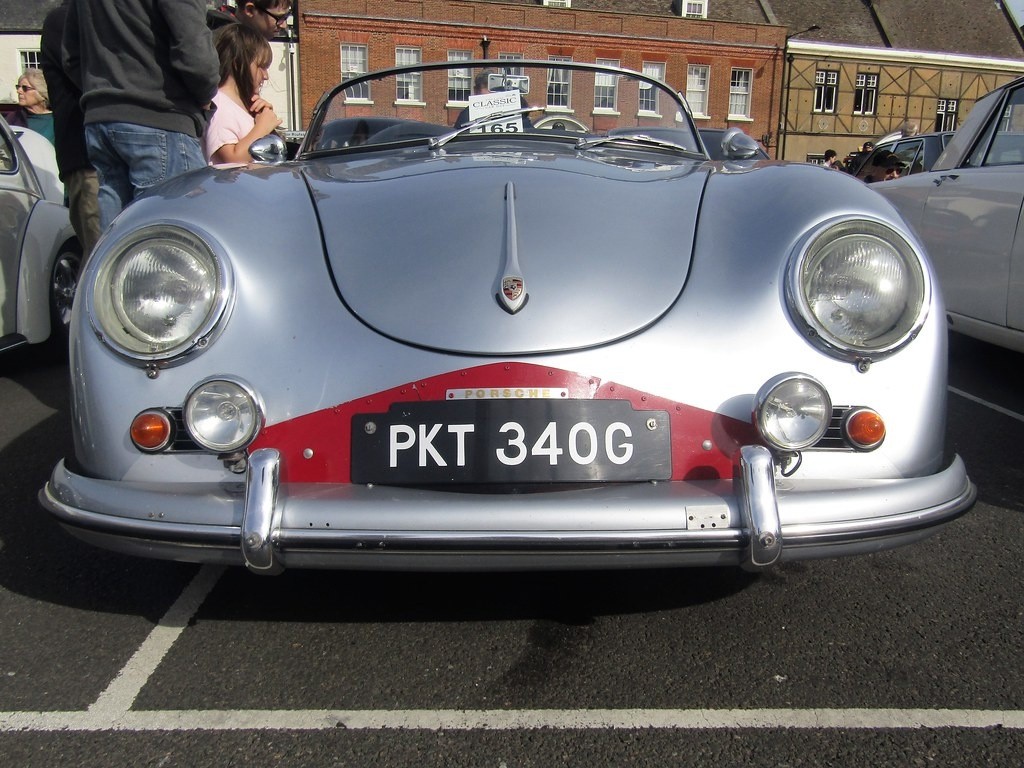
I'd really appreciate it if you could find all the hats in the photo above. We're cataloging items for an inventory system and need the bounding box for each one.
[863,142,874,149]
[872,150,906,168]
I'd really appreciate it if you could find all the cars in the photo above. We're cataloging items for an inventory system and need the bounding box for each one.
[851,75,1024,365]
[0,110,86,370]
[32,57,978,580]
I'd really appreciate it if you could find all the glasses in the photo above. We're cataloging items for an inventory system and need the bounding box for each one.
[879,166,903,174]
[15,85,35,92]
[253,2,292,26]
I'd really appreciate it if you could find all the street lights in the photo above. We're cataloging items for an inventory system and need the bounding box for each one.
[774,24,824,163]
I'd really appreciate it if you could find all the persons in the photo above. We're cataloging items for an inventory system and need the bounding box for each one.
[8,0,295,285]
[453,68,536,134]
[819,120,918,184]
